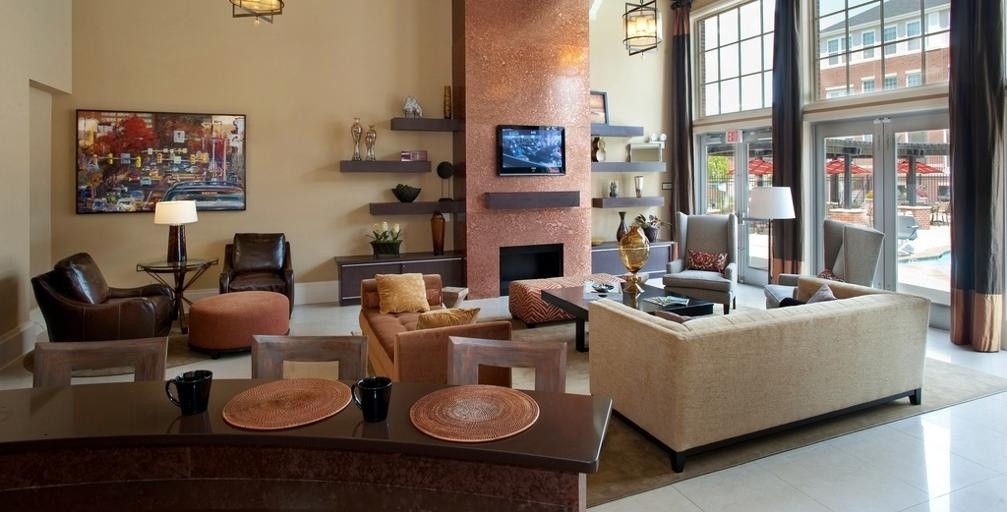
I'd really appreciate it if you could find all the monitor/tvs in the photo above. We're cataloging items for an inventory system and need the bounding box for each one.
[496,124,567,177]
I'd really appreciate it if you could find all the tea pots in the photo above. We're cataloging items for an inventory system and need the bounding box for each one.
[593,284,615,292]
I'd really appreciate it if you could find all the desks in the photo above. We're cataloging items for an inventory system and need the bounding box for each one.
[0,378,612,512]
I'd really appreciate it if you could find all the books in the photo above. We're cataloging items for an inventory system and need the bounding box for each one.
[644,295,690,307]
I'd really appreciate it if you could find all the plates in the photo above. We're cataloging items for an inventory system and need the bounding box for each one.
[593,240,607,246]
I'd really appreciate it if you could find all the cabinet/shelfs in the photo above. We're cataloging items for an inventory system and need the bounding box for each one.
[333,250,462,308]
[592,238,675,279]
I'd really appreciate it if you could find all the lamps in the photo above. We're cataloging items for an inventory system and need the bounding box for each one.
[152,200,200,262]
[646,134,667,149]
[230,0,284,30]
[621,0,665,56]
[748,186,797,285]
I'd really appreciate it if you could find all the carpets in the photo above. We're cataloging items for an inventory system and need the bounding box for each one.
[23,313,210,379]
[586,355,1007,509]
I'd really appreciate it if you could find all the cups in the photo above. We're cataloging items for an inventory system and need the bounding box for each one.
[353,378,390,424]
[165,369,212,419]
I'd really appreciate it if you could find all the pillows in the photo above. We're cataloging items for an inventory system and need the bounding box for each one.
[374,273,431,314]
[817,270,847,283]
[807,281,837,303]
[415,307,481,330]
[686,248,728,274]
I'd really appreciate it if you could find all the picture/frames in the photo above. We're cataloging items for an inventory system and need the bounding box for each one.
[75,108,247,215]
[590,90,609,124]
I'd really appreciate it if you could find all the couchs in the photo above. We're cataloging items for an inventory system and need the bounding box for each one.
[186,290,291,359]
[764,219,886,309]
[507,272,628,329]
[219,231,296,321]
[588,276,931,473]
[31,253,173,341]
[661,212,738,315]
[357,273,513,389]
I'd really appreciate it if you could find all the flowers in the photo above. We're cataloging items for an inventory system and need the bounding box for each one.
[629,213,673,231]
[365,221,402,242]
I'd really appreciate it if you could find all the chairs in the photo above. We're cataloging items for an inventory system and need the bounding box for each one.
[896,215,919,256]
[250,333,369,381]
[32,338,170,389]
[446,333,569,394]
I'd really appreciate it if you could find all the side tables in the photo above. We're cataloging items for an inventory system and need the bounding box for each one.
[134,259,218,334]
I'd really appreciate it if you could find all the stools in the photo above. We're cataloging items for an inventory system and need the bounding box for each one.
[442,285,470,310]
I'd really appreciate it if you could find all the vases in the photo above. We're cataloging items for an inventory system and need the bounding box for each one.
[616,211,628,241]
[643,228,658,242]
[350,117,363,160]
[431,211,445,256]
[364,131,372,160]
[368,124,377,161]
[369,239,402,258]
